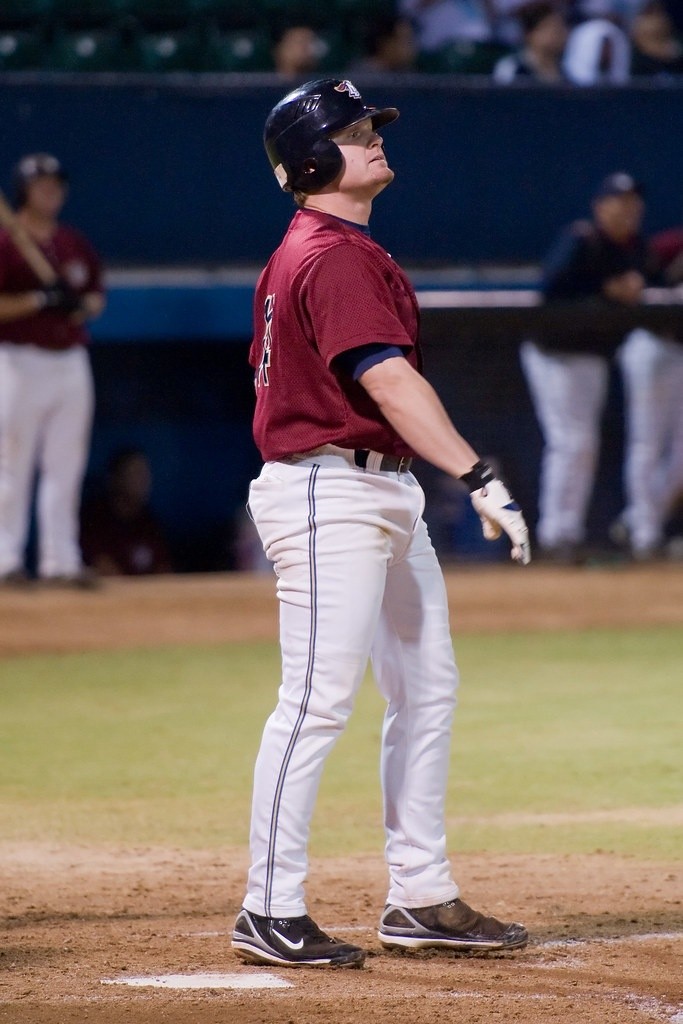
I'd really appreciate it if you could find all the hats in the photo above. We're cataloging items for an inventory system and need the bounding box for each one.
[594,173,647,203]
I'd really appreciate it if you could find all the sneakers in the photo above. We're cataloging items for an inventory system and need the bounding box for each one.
[231,905,366,971]
[375,895,530,956]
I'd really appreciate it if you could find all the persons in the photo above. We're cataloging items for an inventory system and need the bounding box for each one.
[0,0,683,83]
[0,151,183,588]
[517,176,683,557]
[230,76,531,968]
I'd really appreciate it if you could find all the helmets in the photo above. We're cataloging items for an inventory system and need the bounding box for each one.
[6,151,72,212]
[262,78,401,194]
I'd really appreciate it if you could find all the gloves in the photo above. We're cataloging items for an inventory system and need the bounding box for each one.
[462,458,533,567]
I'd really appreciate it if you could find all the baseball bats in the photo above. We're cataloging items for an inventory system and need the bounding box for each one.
[0,200,62,288]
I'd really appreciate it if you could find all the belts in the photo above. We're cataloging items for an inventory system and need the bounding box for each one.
[355,450,414,474]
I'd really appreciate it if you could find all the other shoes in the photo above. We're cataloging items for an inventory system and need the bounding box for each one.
[600,530,655,568]
[544,544,586,570]
[1,569,40,593]
[49,568,97,593]
